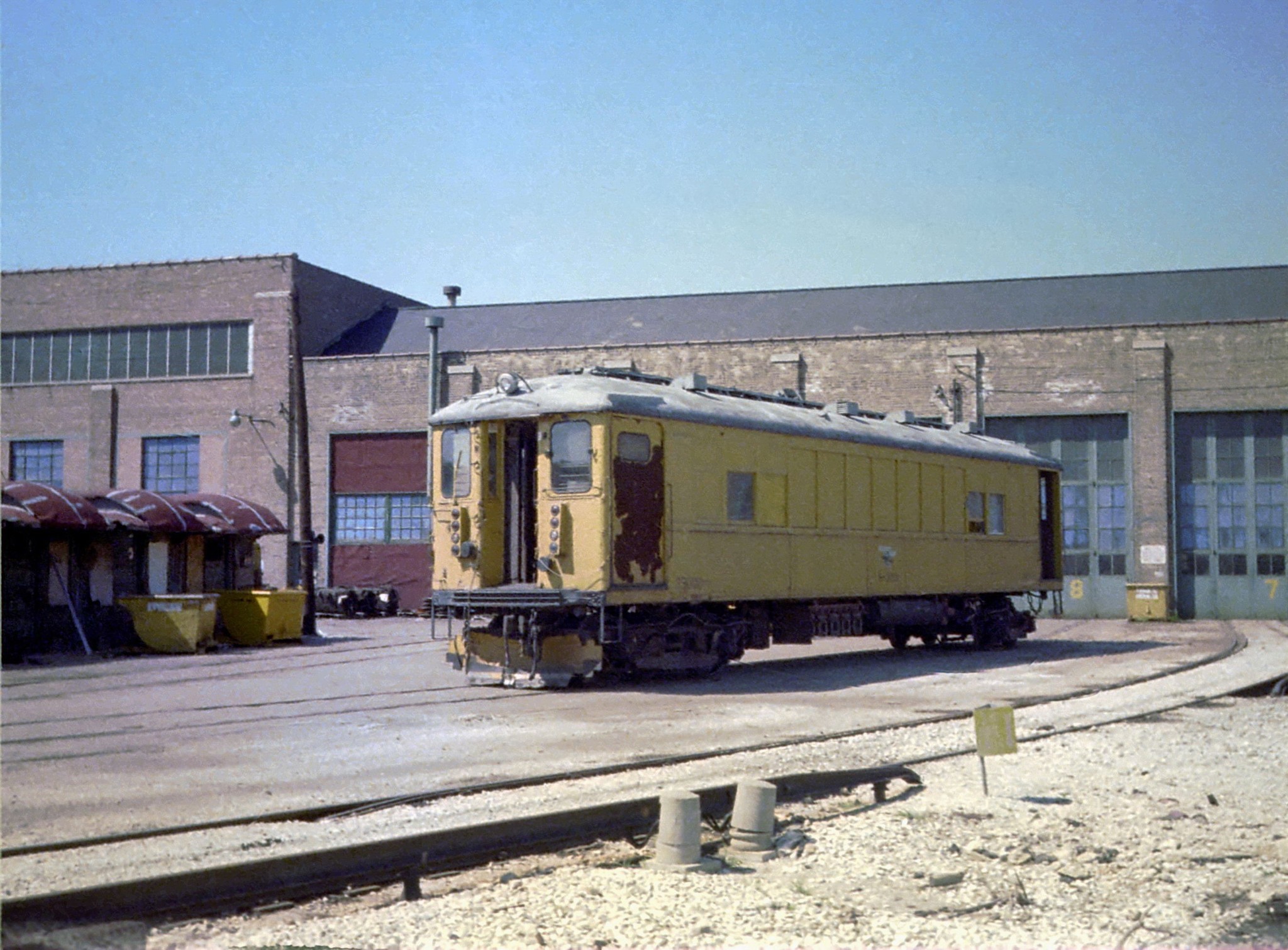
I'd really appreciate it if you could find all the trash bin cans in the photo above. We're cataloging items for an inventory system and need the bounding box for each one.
[207,590,308,647]
[119,594,221,655]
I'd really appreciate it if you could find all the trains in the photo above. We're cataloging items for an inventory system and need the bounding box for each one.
[424,356,1067,688]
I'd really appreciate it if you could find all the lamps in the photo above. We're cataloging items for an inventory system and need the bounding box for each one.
[229,409,254,428]
